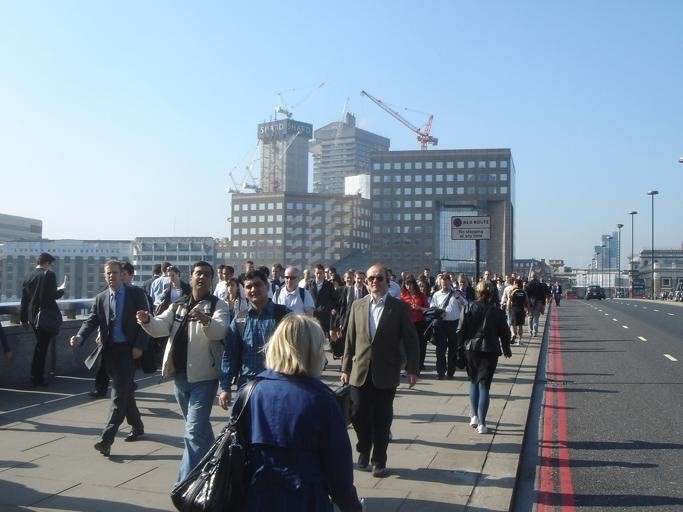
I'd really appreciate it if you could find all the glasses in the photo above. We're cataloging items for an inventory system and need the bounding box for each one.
[367,276,384,282]
[284,276,296,280]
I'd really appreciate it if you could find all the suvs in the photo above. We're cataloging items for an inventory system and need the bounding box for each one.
[585,286,601,300]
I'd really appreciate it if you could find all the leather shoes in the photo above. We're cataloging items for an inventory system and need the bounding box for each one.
[124,428,145,441]
[358,450,370,468]
[95,441,111,456]
[372,461,386,477]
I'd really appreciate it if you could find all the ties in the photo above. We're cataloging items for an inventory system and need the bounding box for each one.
[110,292,118,322]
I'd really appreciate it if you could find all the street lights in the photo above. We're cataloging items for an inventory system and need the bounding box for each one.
[628,211,638,298]
[677,157,682,164]
[600,245,605,288]
[616,222,624,298]
[583,258,596,288]
[606,236,614,297]
[595,252,599,285]
[647,190,658,299]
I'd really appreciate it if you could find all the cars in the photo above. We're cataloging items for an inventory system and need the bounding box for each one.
[673,282,682,302]
[615,289,626,297]
[599,287,605,299]
[644,287,665,299]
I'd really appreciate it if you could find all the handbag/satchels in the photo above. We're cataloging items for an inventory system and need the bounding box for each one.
[335,383,352,425]
[452,304,470,369]
[424,317,438,346]
[330,337,345,360]
[35,307,60,336]
[141,342,164,373]
[171,379,260,512]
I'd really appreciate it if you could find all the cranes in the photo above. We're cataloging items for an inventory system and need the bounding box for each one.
[359,89,438,151]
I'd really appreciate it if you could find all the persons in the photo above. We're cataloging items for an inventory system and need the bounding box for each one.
[218,271,292,412]
[20,252,66,387]
[137,262,230,488]
[230,314,368,511]
[0,322,11,361]
[340,264,419,476]
[456,281,511,434]
[88,261,561,398]
[68,259,148,458]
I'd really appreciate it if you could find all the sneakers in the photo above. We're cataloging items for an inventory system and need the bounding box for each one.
[469,416,478,427]
[475,425,488,434]
